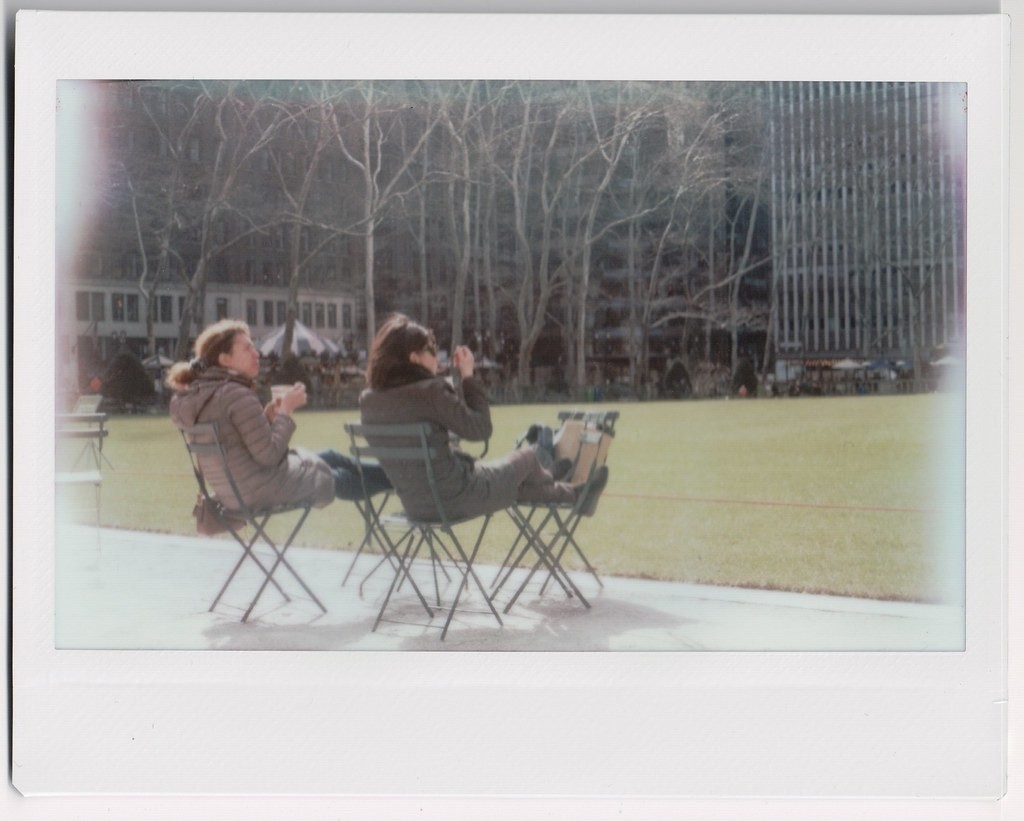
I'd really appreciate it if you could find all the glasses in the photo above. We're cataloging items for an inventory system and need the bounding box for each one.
[413,344,437,357]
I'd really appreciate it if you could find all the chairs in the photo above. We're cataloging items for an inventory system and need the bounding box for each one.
[54,407,109,525]
[341,405,620,640]
[170,418,329,626]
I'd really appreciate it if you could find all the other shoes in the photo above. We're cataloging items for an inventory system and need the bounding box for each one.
[550,456,572,483]
[573,466,608,516]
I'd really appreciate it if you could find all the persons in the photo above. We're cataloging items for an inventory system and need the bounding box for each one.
[170,318,397,517]
[358,313,609,519]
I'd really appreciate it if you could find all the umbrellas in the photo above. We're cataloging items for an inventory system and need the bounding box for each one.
[261,319,342,358]
[435,349,500,372]
[834,357,964,371]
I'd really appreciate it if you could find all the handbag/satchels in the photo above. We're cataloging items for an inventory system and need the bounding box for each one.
[191,494,248,535]
